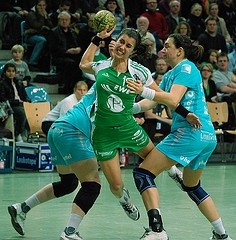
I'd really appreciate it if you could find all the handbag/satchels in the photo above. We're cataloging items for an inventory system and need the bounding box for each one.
[0,100,14,122]
[24,85,52,103]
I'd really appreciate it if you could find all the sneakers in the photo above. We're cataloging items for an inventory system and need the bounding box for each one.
[8,203,27,236]
[167,168,186,192]
[209,230,232,240]
[139,226,169,240]
[119,188,140,221]
[60,226,87,240]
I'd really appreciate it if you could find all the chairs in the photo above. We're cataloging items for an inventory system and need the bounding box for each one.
[21,21,236,163]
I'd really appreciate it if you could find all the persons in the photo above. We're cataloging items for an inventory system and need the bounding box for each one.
[125,33,231,240]
[0,0,236,145]
[80,25,203,221]
[6,80,100,239]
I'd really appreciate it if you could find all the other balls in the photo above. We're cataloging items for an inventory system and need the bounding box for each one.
[94,9,115,33]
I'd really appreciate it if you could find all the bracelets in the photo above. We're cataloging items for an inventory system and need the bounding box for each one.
[91,35,103,48]
[141,87,156,101]
[132,103,141,115]
[174,105,190,119]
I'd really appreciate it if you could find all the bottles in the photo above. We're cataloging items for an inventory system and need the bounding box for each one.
[119,152,129,167]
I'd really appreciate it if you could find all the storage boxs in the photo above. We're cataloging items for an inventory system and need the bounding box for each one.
[15,142,54,172]
